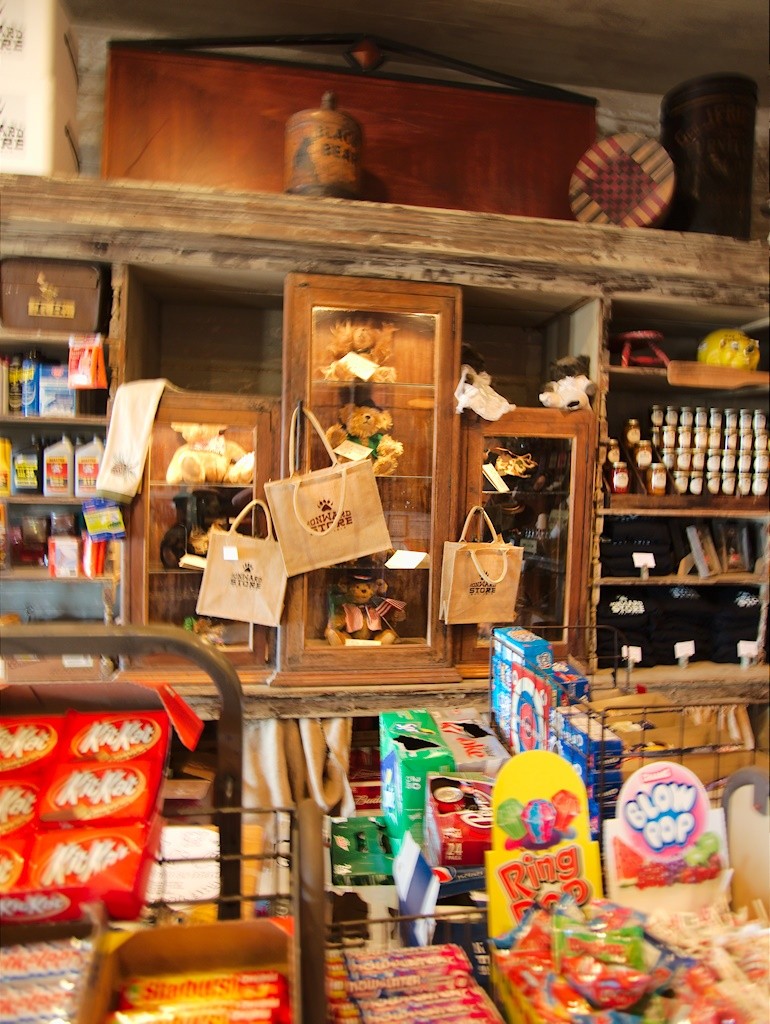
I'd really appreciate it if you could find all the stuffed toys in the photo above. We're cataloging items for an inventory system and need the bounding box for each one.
[159,488,242,569]
[324,570,406,645]
[455,342,516,422]
[325,402,404,476]
[165,421,256,485]
[538,353,598,411]
[187,619,228,650]
[320,316,398,382]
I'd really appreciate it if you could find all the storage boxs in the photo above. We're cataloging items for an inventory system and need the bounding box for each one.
[379,625,626,934]
[0,682,296,1024]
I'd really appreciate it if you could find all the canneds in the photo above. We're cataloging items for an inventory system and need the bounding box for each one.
[607,405,770,495]
[434,786,466,813]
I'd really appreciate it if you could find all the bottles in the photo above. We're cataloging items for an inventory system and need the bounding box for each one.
[0,349,41,422]
[12,433,108,498]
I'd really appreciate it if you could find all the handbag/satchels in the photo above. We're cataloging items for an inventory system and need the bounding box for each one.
[438,508,524,625]
[264,405,393,579]
[195,500,286,628]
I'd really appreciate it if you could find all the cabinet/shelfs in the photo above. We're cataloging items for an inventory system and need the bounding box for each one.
[0,175,770,720]
